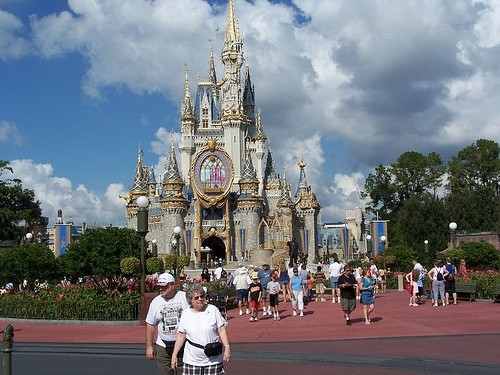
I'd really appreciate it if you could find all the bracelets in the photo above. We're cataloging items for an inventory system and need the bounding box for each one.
[224,344,230,347]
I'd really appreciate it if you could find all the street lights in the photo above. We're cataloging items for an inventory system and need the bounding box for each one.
[171,226,182,276]
[380,235,386,267]
[449,221,458,239]
[366,235,373,257]
[424,239,430,251]
[136,195,149,294]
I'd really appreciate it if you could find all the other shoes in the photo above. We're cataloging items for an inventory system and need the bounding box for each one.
[409,299,457,306]
[347,319,352,325]
[239,296,309,321]
[338,300,340,303]
[332,300,335,303]
[365,321,370,324]
[321,298,326,301]
[316,298,318,301]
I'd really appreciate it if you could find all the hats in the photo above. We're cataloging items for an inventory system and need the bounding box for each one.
[262,263,269,266]
[446,257,451,262]
[156,272,174,286]
[239,267,247,273]
[252,275,258,279]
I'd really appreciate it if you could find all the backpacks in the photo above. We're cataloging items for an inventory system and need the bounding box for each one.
[434,267,443,280]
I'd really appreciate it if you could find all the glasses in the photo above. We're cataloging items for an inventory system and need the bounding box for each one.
[190,294,204,300]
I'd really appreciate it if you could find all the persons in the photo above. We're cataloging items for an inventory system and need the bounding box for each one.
[443,257,457,304]
[179,267,187,280]
[428,260,449,307]
[201,268,210,282]
[329,256,344,303]
[171,283,231,375]
[145,273,190,375]
[378,265,386,293]
[337,264,375,325]
[233,263,291,322]
[287,236,299,266]
[405,256,427,307]
[366,258,379,293]
[288,261,314,316]
[214,263,227,280]
[460,259,467,275]
[311,266,326,302]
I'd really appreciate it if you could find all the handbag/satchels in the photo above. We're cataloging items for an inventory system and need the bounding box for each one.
[160,339,186,356]
[186,339,222,356]
[447,273,454,280]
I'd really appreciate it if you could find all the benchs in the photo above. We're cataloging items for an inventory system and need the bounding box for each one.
[425,281,476,302]
